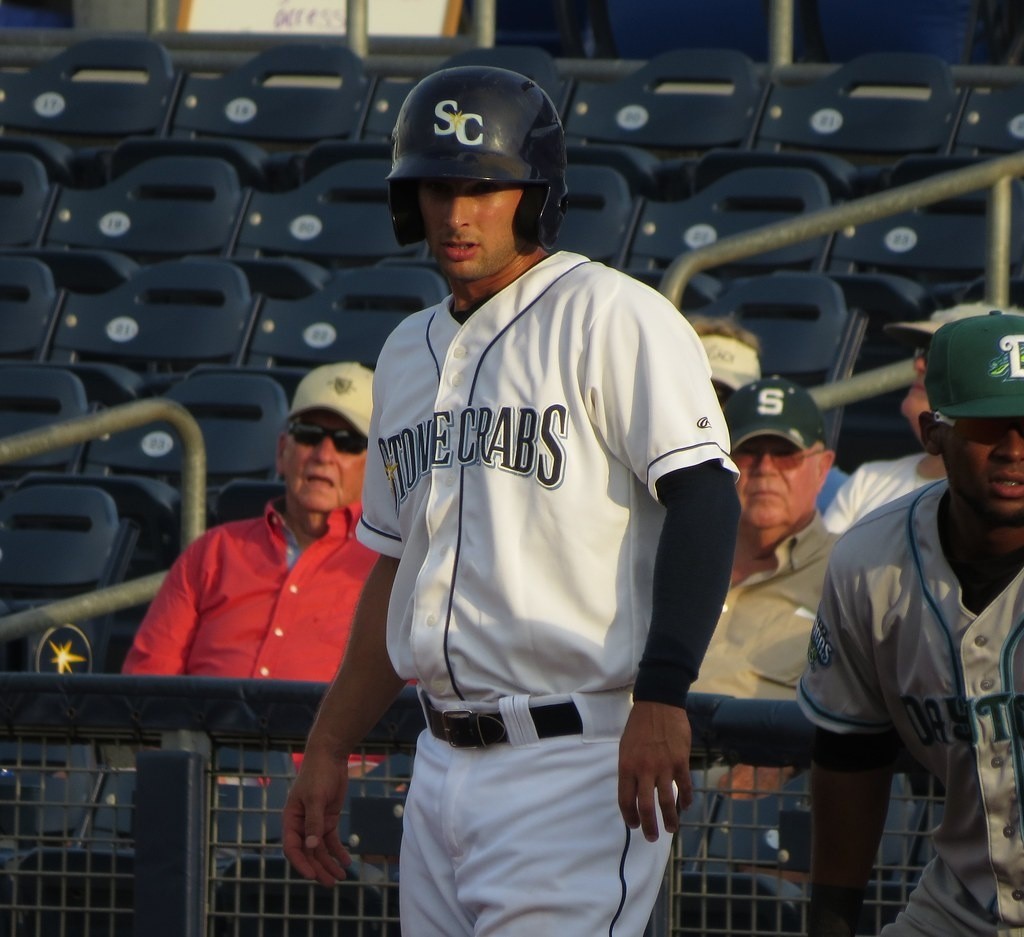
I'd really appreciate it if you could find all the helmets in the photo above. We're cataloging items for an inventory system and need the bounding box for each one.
[386,66,568,251]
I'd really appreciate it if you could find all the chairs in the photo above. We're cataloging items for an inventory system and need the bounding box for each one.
[0,35,1024,937]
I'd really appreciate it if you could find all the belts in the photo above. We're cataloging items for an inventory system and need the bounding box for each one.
[420,689,583,750]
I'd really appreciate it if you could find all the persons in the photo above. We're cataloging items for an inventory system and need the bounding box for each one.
[282,66,742,937]
[683,378,846,797]
[686,314,850,516]
[797,307,1024,937]
[822,303,1024,534]
[120,362,424,785]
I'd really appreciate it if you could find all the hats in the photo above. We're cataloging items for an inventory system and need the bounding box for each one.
[924,310,1024,419]
[293,361,374,440]
[721,377,827,455]
[697,333,760,393]
[884,302,1024,345]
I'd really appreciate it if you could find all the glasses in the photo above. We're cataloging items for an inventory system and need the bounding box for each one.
[914,338,930,360]
[732,444,822,470]
[287,422,368,455]
[932,411,1024,445]
